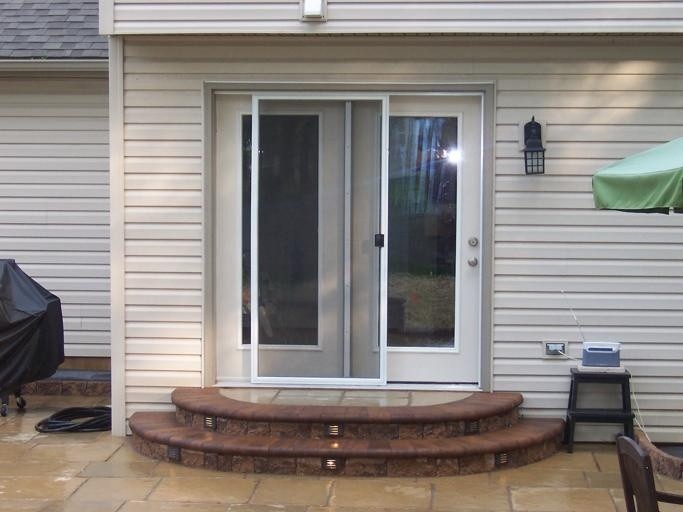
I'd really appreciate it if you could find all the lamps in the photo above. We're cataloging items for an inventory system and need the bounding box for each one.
[518,116,547,177]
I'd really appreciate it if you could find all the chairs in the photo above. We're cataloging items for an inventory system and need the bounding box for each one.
[615,434,683,511]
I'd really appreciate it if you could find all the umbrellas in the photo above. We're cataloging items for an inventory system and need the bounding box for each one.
[591,135,683,218]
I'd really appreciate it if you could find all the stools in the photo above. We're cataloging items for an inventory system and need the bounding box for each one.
[564,367,636,455]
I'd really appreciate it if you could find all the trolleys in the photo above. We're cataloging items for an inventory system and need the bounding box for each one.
[0,258,61,417]
